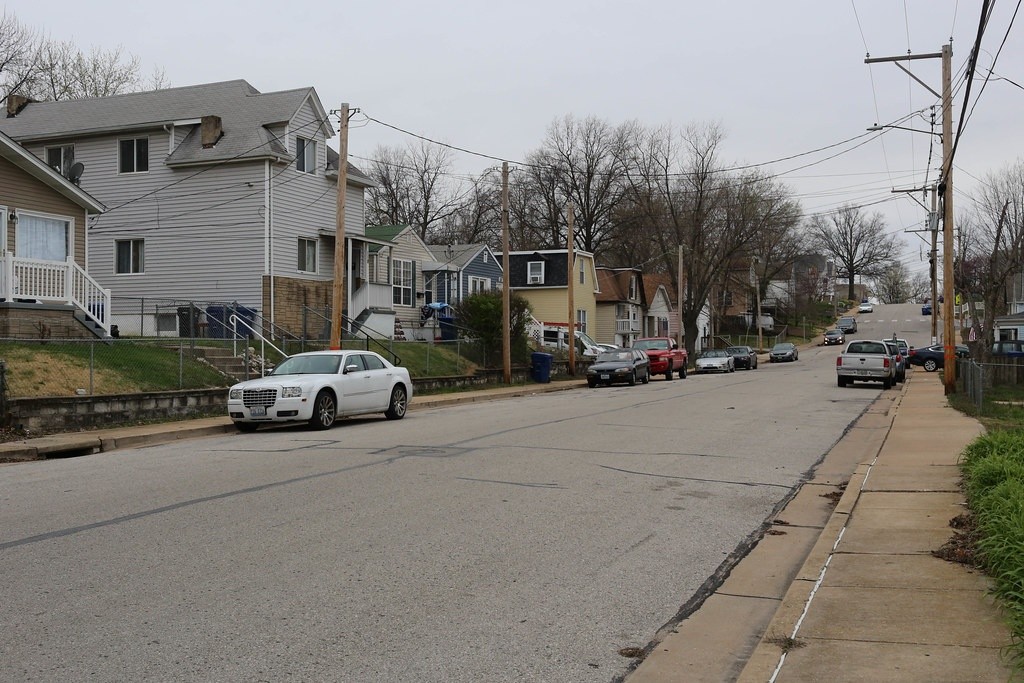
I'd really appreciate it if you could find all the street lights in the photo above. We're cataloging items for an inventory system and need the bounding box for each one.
[867,122,956,397]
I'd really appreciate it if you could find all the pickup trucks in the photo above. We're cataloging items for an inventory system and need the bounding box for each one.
[836,340,899,390]
[628,336,688,381]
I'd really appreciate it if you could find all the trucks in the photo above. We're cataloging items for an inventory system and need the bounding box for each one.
[522,320,604,369]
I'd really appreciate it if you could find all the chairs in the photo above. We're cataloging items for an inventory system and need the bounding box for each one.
[899,343,904,347]
[874,347,883,352]
[854,346,862,352]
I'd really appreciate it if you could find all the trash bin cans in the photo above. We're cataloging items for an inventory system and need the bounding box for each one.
[206,305,257,338]
[438,317,458,343]
[177,306,199,338]
[531,352,552,383]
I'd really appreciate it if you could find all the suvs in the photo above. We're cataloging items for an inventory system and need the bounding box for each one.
[835,316,857,334]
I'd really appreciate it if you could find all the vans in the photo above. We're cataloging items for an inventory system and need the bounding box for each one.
[881,339,915,369]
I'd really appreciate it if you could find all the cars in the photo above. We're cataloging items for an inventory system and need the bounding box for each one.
[227,349,414,433]
[908,343,970,373]
[694,348,735,373]
[885,342,907,383]
[587,347,651,389]
[858,302,873,313]
[725,345,758,370]
[922,304,933,315]
[823,329,845,346]
[769,342,798,363]
